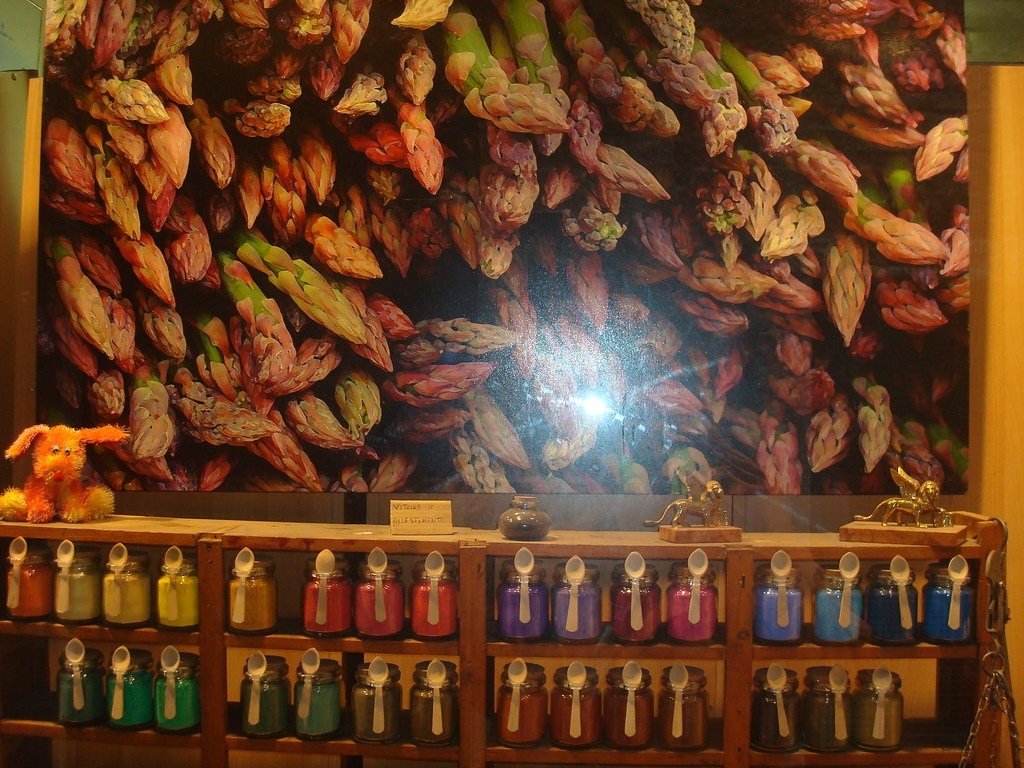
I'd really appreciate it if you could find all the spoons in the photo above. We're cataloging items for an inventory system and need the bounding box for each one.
[232,544,251,624]
[688,548,705,625]
[238,649,446,734]
[369,548,389,619]
[949,554,968,630]
[626,551,651,630]
[515,545,536,622]
[892,555,915,628]
[162,646,182,720]
[162,546,180,624]
[65,636,88,707]
[110,646,131,720]
[763,662,892,739]
[506,658,687,738]
[773,548,793,627]
[106,542,127,616]
[838,550,861,626]
[566,555,586,630]
[9,538,28,609]
[313,550,337,623]
[49,539,77,614]
[422,550,447,624]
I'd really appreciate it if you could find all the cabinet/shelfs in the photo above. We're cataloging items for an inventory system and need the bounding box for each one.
[2,513,1015,768]
